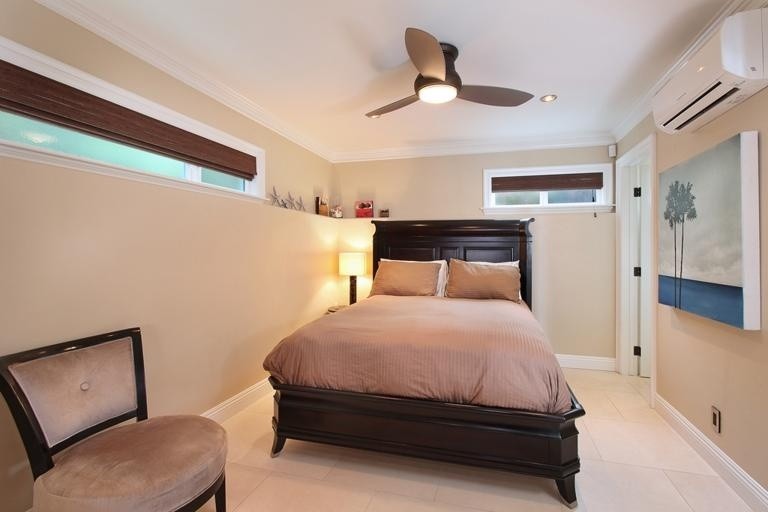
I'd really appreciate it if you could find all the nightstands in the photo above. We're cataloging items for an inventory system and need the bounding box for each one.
[325,305,344,314]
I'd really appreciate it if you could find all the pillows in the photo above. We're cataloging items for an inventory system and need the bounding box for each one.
[368,260,440,298]
[379,257,447,296]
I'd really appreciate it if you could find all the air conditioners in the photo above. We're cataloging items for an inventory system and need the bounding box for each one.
[651,7,767,136]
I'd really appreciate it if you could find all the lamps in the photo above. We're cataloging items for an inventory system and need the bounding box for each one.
[412,72,462,104]
[338,253,366,306]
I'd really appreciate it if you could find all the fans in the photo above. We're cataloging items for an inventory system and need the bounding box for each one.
[364,28,532,121]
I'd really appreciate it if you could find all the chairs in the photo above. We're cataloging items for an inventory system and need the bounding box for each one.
[1,327,229,512]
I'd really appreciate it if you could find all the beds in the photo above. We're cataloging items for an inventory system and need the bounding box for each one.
[260,218,584,510]
